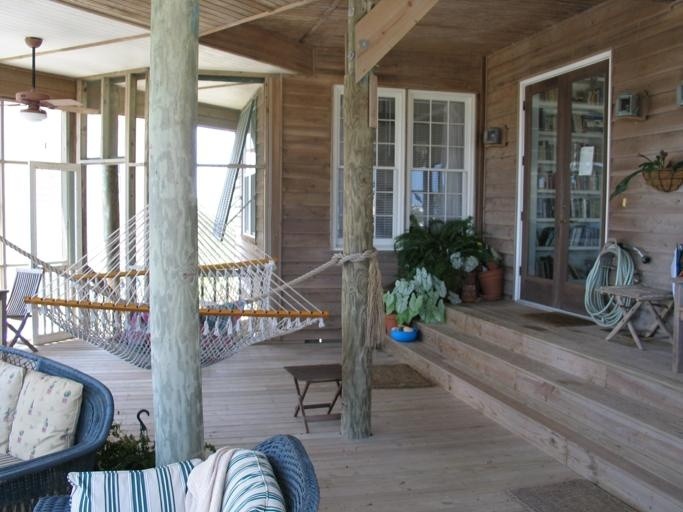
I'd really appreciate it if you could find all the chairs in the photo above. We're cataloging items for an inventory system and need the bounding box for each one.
[33,435,320,512]
[5,269,46,352]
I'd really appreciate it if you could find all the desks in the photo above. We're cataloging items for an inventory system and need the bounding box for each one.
[0,290,10,346]
[672,277,683,373]
[595,284,673,351]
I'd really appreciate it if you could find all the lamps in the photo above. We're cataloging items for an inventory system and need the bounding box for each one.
[19,101,47,121]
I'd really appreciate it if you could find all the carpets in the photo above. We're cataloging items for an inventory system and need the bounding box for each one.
[370,363,433,389]
[507,477,637,512]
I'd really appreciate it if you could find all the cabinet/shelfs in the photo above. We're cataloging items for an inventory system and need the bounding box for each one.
[528,73,606,284]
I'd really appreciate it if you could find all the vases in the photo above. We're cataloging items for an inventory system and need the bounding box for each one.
[462,261,503,303]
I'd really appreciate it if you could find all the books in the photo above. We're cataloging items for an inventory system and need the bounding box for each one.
[536,85,604,280]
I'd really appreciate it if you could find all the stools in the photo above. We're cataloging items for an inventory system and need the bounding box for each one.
[283,363,342,433]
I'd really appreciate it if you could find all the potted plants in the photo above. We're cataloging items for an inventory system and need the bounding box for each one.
[382,267,462,337]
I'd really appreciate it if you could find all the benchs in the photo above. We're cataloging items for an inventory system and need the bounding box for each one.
[0,344,114,505]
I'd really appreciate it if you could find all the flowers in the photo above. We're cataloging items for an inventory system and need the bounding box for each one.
[609,150,683,201]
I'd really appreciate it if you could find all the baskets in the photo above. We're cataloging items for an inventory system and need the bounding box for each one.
[641,167,683,192]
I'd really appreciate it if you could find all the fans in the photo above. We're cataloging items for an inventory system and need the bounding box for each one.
[4,36,99,121]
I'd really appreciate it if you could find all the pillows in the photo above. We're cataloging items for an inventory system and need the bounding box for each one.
[221,449,286,512]
[67,457,205,512]
[9,369,84,461]
[0,359,26,457]
